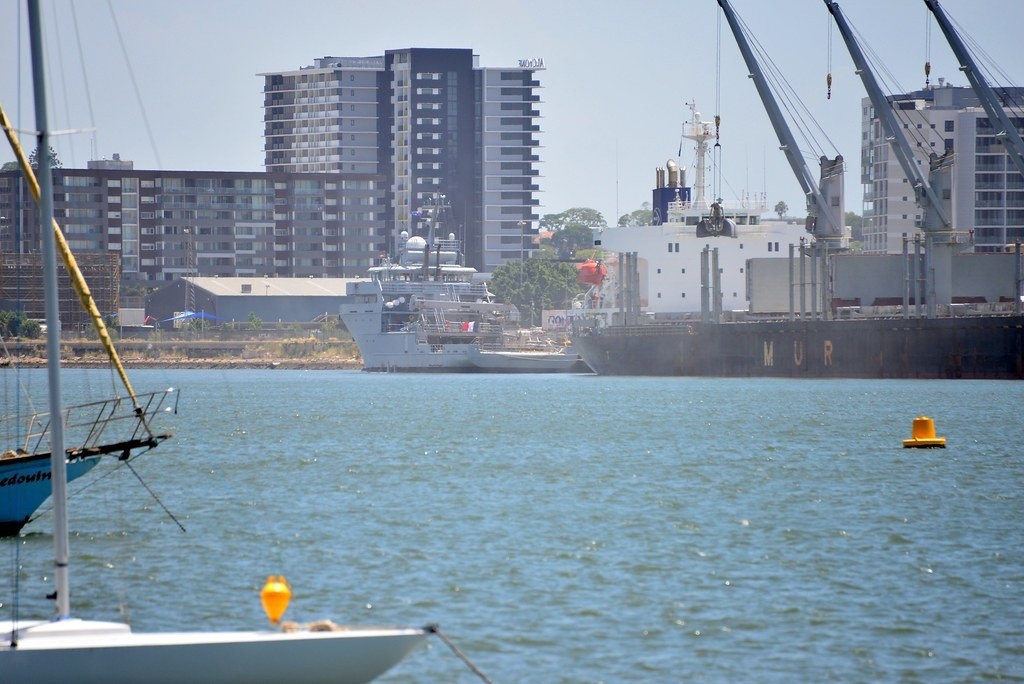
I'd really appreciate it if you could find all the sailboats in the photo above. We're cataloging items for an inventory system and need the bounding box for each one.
[1,1,440,684]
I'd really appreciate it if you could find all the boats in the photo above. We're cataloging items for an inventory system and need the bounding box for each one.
[566,0,1024,380]
[0,104,177,536]
[336,96,855,370]
